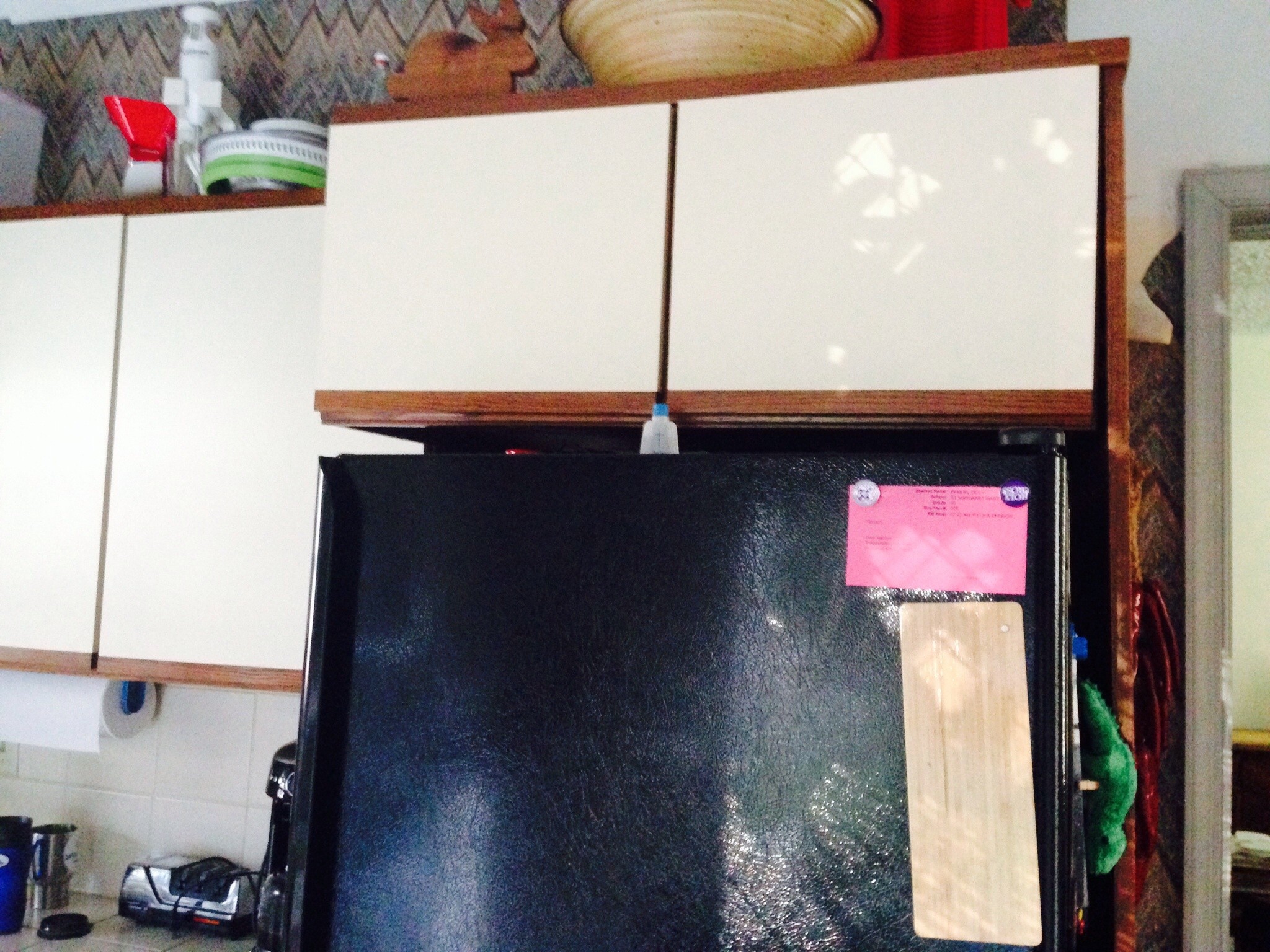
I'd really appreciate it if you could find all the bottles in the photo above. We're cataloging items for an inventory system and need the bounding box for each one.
[639,404,678,454]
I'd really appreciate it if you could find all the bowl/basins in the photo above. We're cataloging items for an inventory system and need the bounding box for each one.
[558,0,883,85]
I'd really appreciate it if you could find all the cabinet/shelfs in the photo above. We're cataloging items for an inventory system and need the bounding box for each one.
[0,203,421,697]
[311,35,1131,426]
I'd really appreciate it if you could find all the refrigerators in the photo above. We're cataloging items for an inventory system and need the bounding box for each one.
[273,426,1072,952]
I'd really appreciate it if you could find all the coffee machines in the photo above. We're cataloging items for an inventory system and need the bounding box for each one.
[252,741,298,952]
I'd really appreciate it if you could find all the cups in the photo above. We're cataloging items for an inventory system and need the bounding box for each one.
[0,816,33,934]
[29,824,78,910]
